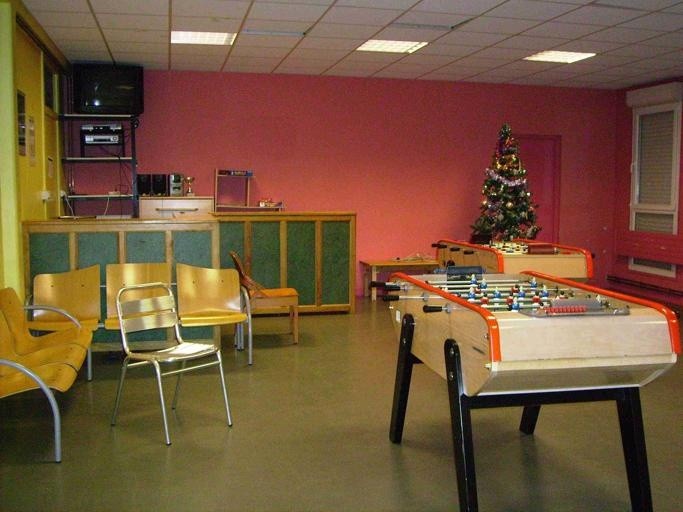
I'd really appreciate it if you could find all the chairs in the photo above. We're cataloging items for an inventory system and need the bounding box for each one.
[229,251,300,344]
[111,282,233,446]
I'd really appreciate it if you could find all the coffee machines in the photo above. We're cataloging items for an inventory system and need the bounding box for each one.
[137,174,151,198]
[168,174,184,197]
[152,174,165,197]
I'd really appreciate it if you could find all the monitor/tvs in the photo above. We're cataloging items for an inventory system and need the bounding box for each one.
[72,62,144,119]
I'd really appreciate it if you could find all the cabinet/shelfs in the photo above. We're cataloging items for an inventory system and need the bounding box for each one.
[58,113,138,219]
[139,199,215,220]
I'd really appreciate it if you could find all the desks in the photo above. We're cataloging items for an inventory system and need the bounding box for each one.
[360,260,440,300]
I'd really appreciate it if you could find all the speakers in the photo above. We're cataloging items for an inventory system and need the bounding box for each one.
[136,175,151,194]
[151,174,166,197]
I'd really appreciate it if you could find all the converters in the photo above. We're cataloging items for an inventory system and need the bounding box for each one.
[107,191,120,195]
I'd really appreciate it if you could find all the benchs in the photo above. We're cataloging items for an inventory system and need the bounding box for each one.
[25,262,253,365]
[0,287,94,464]
